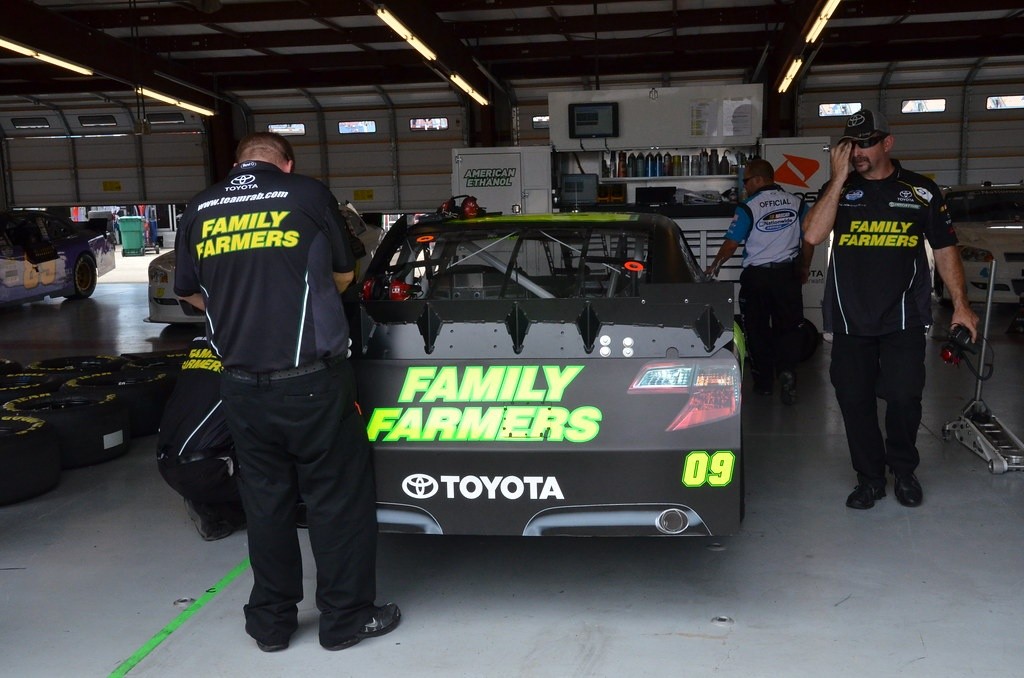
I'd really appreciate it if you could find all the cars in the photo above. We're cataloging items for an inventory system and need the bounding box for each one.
[933,182,1024,303]
[0,211,98,303]
[345,213,746,538]
[144,249,206,324]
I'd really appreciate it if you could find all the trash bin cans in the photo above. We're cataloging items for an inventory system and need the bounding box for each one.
[118,216,145,256]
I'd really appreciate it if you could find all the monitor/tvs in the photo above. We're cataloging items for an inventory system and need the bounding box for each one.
[568,102,619,138]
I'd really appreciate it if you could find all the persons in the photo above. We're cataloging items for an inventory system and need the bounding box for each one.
[798,108,981,511]
[174,133,402,654]
[704,160,813,410]
[155,328,310,541]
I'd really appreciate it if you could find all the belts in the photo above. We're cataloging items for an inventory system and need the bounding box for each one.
[155,450,223,464]
[756,263,797,269]
[218,353,344,381]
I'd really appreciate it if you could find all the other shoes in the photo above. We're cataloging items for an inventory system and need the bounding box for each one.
[778,368,804,405]
[751,372,772,394]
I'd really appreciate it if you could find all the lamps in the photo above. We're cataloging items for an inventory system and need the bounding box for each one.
[0,37,218,116]
[374,4,490,105]
[777,0,841,93]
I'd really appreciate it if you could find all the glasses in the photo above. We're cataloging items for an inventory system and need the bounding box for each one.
[841,134,888,150]
[742,175,759,185]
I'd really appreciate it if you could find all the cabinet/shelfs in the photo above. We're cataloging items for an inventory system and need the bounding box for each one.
[600,149,746,183]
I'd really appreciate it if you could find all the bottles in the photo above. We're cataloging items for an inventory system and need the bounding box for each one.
[609,148,760,203]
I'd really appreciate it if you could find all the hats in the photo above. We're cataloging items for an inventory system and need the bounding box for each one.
[837,109,889,146]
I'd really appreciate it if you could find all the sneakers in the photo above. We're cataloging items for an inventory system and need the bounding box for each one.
[888,466,923,507]
[846,476,886,509]
[255,638,289,652]
[322,602,400,651]
[184,497,248,540]
